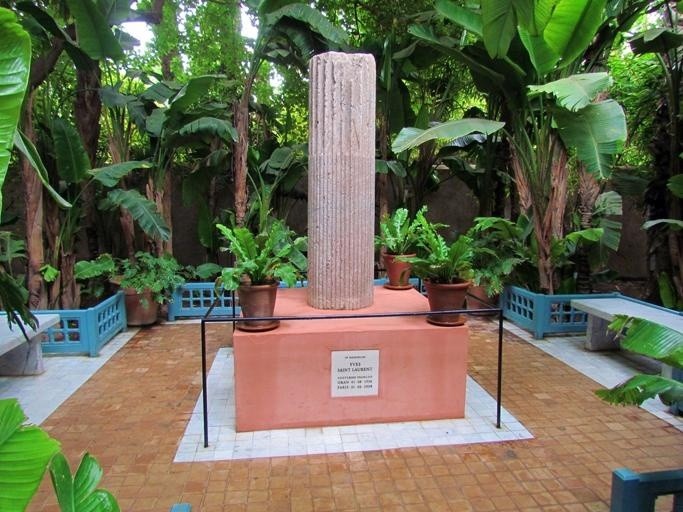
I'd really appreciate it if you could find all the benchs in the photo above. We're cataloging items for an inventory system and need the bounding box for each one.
[567,296,682,383]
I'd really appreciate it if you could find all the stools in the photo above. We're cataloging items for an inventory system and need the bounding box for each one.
[0,310,60,376]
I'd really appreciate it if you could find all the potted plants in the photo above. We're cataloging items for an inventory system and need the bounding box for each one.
[204,214,311,334]
[105,251,186,326]
[373,205,531,329]
